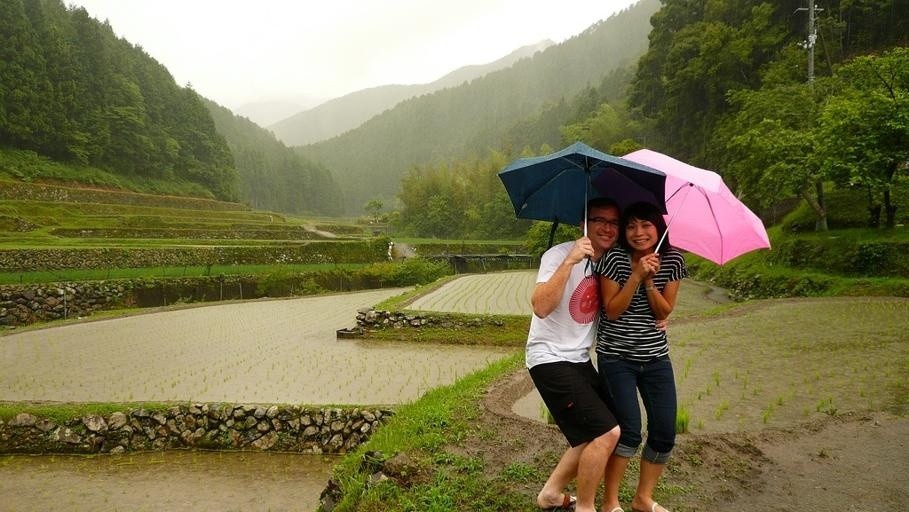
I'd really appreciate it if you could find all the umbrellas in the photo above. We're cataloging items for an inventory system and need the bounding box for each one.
[496,141,771,267]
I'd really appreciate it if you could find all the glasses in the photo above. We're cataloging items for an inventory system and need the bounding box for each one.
[588,216,620,227]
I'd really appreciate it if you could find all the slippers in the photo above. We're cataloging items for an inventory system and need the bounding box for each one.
[536,492,577,512]
[610,507,624,512]
[631,503,670,512]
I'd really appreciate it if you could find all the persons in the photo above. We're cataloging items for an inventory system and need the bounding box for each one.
[594,202,686,512]
[525,197,667,512]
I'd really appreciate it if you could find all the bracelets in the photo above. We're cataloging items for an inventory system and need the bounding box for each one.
[646,285,655,290]
